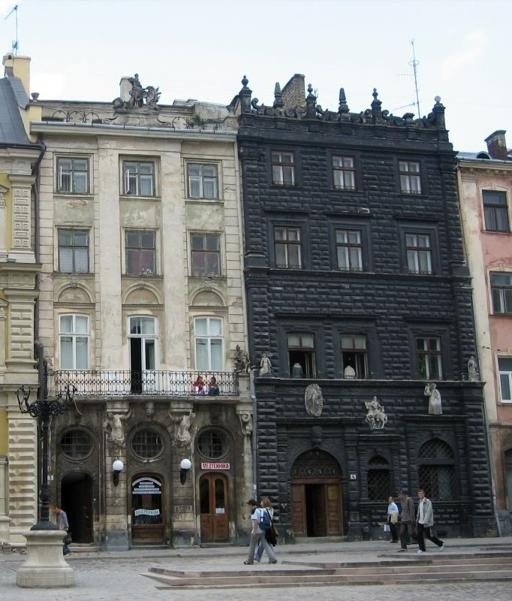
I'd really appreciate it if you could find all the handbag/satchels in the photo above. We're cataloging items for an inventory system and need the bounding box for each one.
[384,524,391,533]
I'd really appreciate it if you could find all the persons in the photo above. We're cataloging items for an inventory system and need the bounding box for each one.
[103,409,132,444]
[51,502,70,556]
[192,376,204,395]
[366,395,385,422]
[254,496,274,562]
[244,499,277,565]
[398,487,418,552]
[260,353,272,373]
[208,377,219,395]
[386,495,399,543]
[468,355,479,377]
[415,488,445,554]
[169,410,196,445]
[425,383,442,416]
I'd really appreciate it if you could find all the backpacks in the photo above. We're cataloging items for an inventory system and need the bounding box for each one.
[257,508,272,530]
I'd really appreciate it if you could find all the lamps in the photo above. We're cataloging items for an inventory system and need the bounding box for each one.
[112,460,124,487]
[180,458,191,485]
